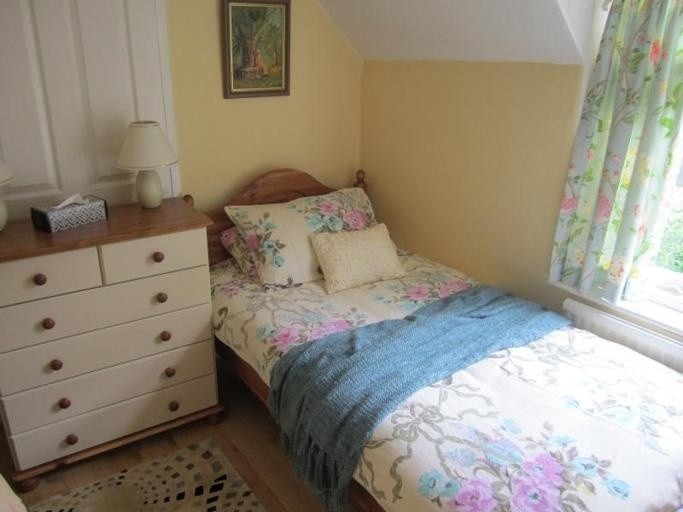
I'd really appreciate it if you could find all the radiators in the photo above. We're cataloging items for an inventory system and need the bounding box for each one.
[562,298,683,374]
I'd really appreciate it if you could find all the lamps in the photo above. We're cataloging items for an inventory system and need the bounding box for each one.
[116,121,181,209]
[0,159,17,232]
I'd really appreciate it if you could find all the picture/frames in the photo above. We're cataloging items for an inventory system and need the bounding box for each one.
[220,0,291,99]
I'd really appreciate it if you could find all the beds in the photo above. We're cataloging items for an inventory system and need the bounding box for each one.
[183,167,683,512]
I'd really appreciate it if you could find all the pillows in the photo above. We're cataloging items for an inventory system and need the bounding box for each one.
[221,226,257,276]
[309,223,409,294]
[224,188,378,293]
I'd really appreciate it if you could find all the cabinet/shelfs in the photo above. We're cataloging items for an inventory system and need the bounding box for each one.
[1,197,226,493]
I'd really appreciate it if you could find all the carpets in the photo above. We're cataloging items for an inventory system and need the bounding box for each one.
[26,426,288,512]
